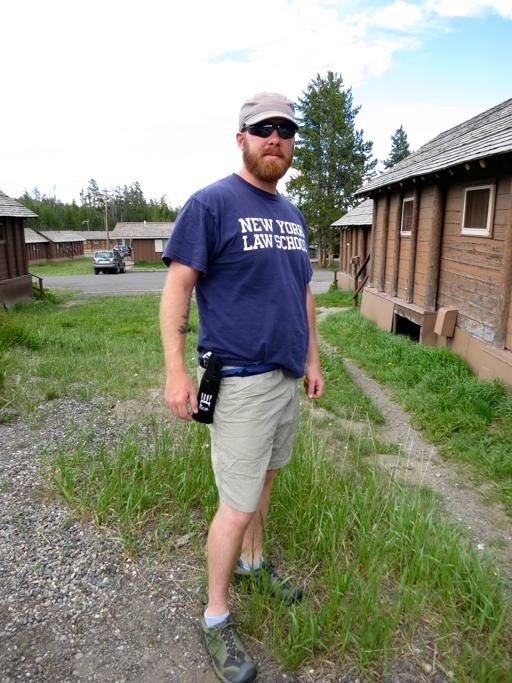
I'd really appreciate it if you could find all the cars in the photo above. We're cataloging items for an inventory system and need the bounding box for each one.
[113,245,131,255]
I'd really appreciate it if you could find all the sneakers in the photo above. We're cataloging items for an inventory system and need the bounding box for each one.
[235,559,302,604]
[199,603,257,682]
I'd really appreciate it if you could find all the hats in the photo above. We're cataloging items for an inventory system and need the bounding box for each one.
[239,92,301,129]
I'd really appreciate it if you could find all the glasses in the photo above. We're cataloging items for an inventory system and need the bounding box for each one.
[241,119,297,139]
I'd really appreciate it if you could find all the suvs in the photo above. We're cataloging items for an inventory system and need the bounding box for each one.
[93,250,127,275]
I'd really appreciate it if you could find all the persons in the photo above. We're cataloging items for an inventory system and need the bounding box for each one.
[156,90,328,682]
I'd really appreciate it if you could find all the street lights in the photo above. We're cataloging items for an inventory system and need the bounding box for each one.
[82,218,89,231]
